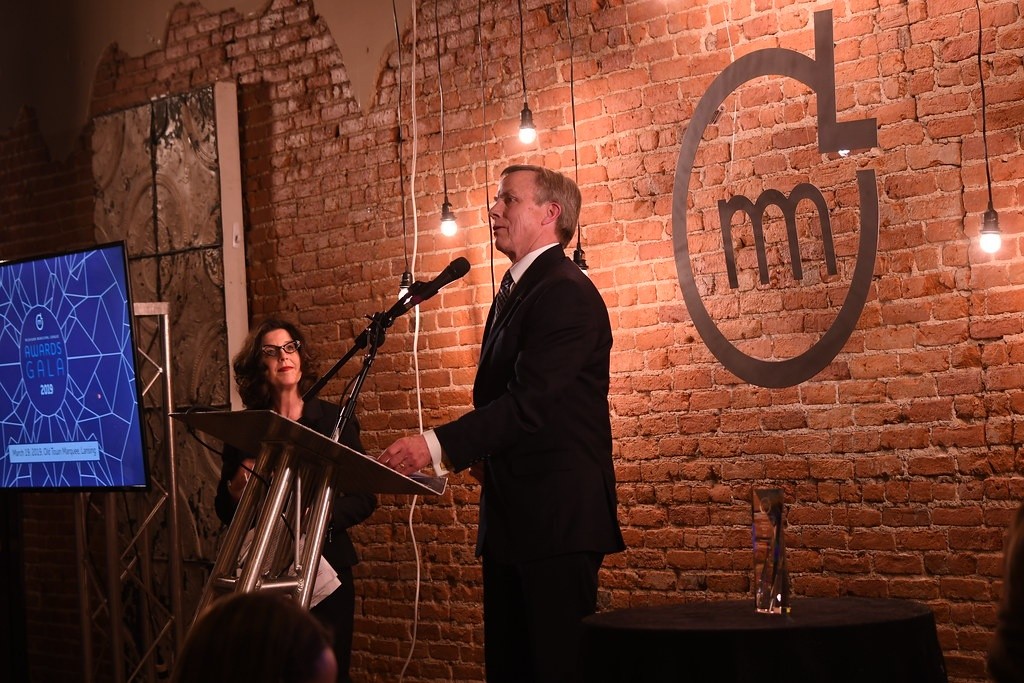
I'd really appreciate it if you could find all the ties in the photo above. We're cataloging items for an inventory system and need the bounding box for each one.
[490,270,514,329]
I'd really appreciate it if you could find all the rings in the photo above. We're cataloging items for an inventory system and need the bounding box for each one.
[399,462,405,468]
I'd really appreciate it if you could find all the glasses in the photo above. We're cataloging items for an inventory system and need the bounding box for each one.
[258,340,301,357]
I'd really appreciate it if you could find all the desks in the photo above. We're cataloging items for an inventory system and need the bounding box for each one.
[574,595,949,683]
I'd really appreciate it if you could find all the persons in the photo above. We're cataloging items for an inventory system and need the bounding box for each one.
[379,164,625,683]
[166,316,379,683]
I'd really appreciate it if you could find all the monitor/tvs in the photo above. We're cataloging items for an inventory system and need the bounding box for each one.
[0,239,152,492]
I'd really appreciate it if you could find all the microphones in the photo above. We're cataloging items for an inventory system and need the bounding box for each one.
[390,257,471,319]
